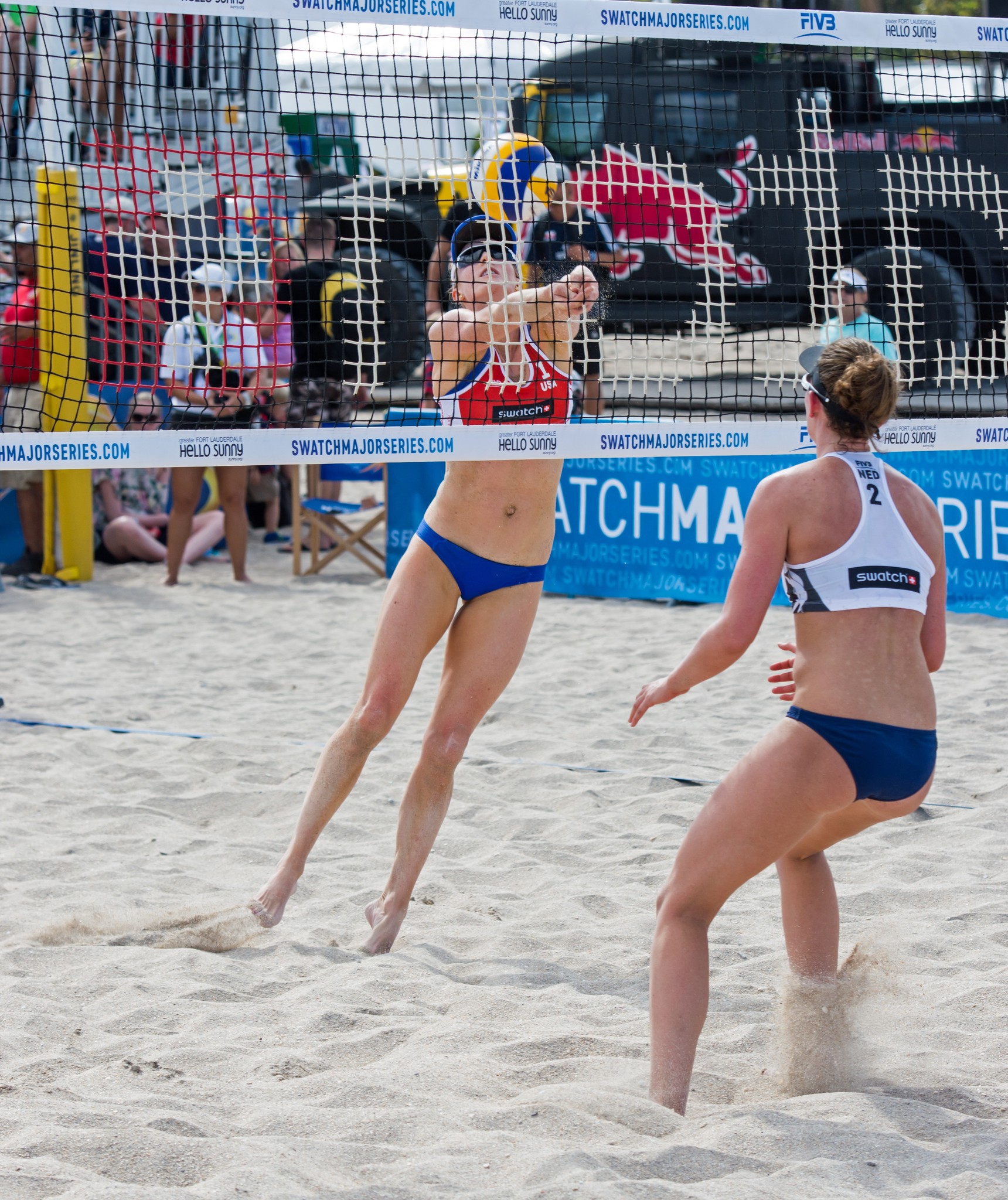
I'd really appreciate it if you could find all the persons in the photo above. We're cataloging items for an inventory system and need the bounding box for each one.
[822,264,898,363]
[421,163,624,416]
[0,0,378,590]
[247,213,599,956]
[627,335,946,1119]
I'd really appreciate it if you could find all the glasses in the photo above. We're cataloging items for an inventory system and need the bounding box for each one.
[193,285,205,293]
[451,243,519,287]
[832,285,855,294]
[802,373,830,404]
[133,414,157,423]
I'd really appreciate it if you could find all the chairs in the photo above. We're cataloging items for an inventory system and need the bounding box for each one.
[292,417,385,578]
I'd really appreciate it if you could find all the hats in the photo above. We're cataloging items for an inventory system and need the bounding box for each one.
[182,263,231,294]
[799,346,827,395]
[451,215,517,277]
[102,196,149,232]
[832,269,867,291]
[1,221,39,244]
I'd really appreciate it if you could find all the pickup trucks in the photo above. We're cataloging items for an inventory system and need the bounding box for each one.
[280,40,1008,390]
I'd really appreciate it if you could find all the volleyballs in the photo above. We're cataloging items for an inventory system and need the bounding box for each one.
[469,131,560,222]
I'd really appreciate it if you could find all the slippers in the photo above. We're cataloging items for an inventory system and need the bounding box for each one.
[6,575,80,590]
[278,541,330,554]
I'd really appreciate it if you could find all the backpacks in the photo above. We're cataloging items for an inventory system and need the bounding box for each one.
[309,263,379,351]
